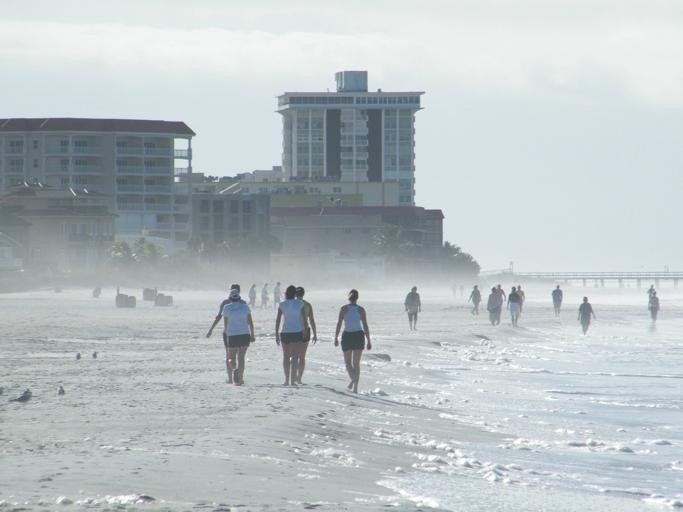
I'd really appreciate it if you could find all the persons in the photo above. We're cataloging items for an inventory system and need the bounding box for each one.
[205,281,318,387]
[404,285,421,331]
[577,296,596,335]
[466,282,526,328]
[335,289,373,393]
[647,284,655,302]
[647,291,661,320]
[551,284,563,315]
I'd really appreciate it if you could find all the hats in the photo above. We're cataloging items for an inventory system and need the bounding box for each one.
[230,288,239,299]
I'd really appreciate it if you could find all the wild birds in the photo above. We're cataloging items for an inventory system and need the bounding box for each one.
[9,388,32,403]
[77,353,82,359]
[58,386,65,395]
[92,351,97,359]
